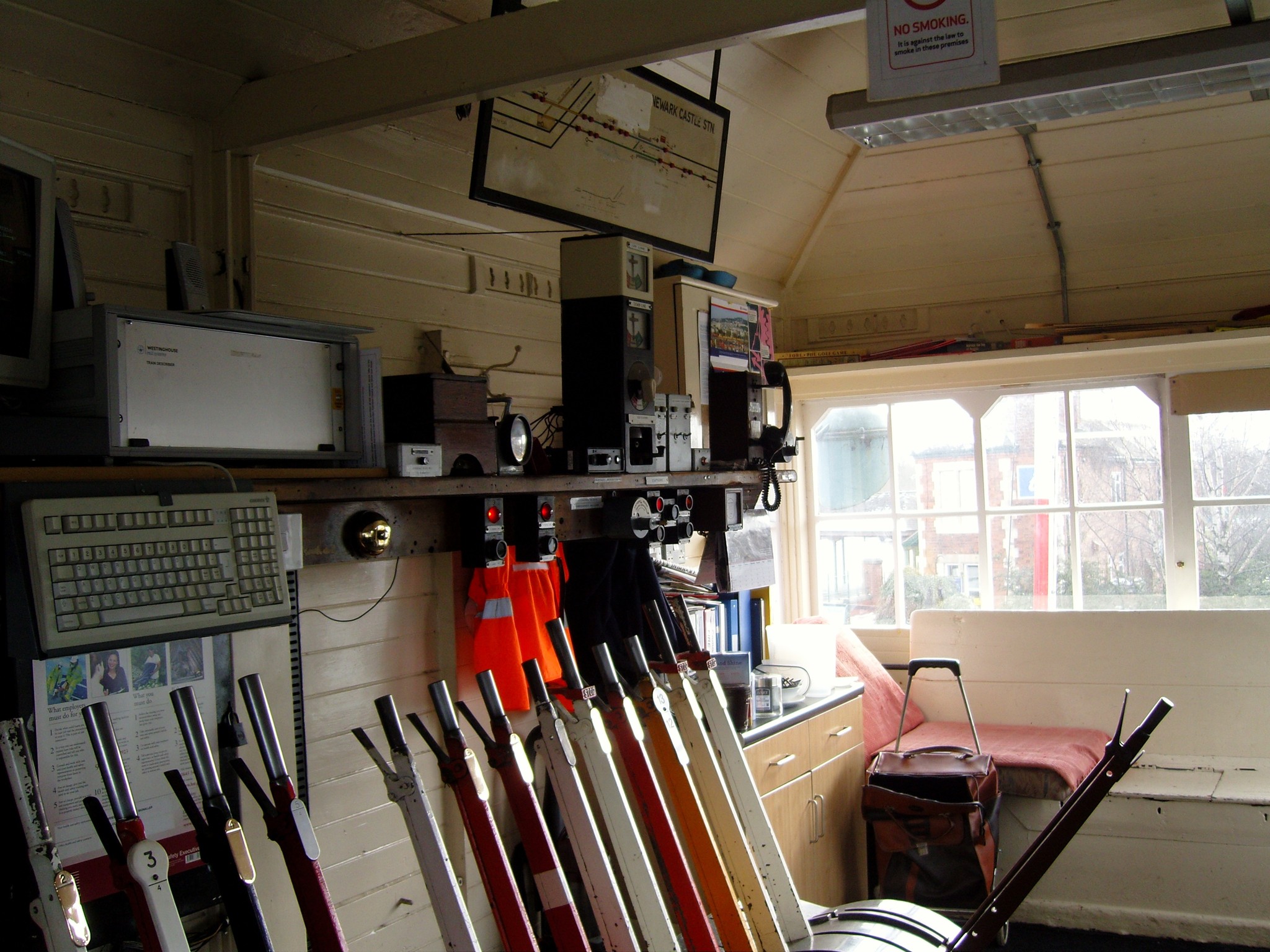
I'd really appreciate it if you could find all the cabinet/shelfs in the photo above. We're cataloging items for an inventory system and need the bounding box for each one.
[728,679,872,905]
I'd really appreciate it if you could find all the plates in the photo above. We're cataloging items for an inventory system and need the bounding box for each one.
[781,695,805,708]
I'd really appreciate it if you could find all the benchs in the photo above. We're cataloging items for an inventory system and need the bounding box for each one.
[900,609,1269,947]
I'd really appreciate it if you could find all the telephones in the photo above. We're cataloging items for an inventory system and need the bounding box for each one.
[708,361,804,511]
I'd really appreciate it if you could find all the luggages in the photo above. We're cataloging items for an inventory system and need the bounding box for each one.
[861,657,1009,945]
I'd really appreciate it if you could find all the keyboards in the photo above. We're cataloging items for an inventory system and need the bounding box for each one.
[21,493,291,650]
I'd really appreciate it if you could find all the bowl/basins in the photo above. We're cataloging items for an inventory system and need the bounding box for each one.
[781,680,801,698]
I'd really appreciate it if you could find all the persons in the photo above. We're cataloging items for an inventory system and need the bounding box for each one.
[47,647,162,702]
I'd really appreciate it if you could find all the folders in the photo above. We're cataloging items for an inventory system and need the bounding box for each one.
[718,589,752,671]
[717,599,738,651]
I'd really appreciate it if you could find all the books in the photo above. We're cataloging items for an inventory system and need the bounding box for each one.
[649,557,726,653]
